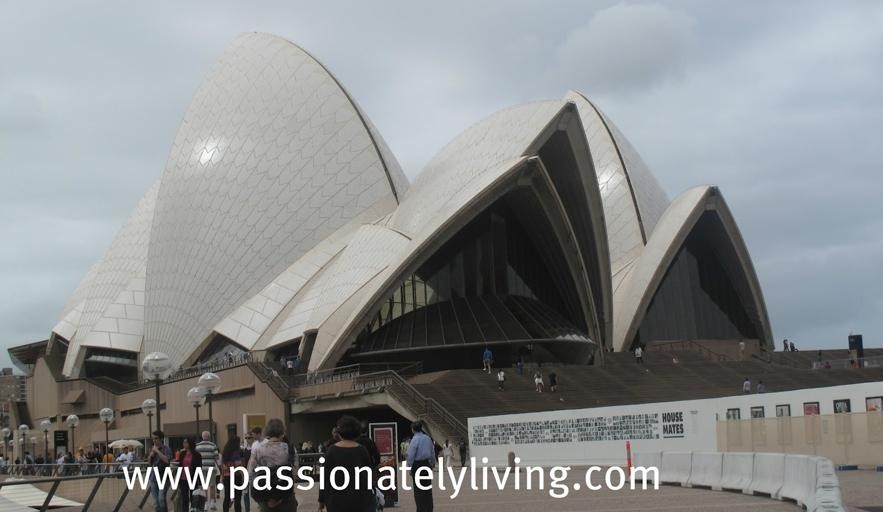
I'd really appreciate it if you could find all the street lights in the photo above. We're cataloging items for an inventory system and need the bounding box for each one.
[187,386,206,443]
[197,372,221,443]
[0,419,53,465]
[98,407,113,462]
[141,351,171,433]
[141,398,157,438]
[65,414,78,461]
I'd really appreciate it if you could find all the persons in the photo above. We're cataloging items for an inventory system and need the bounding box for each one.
[0,339,867,512]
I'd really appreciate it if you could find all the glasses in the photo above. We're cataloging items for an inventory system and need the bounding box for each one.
[244,437,254,440]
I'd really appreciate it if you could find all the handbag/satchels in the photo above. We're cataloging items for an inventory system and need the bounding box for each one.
[250,440,294,501]
